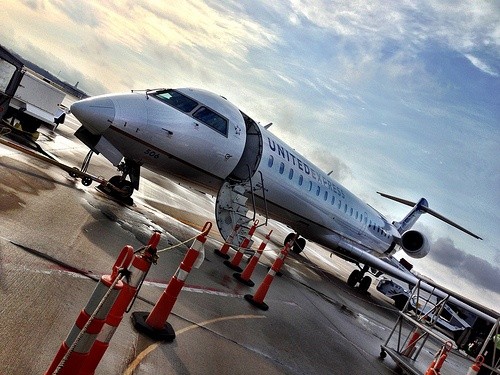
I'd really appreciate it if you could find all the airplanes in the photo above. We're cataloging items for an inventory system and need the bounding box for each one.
[67,87,500,328]
